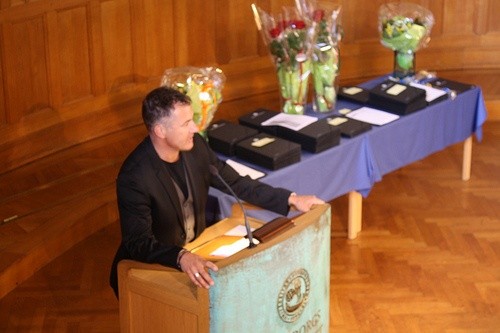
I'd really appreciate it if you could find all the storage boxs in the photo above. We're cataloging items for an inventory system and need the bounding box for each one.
[207,80,426,171]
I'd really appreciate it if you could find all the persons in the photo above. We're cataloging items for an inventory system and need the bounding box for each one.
[109,86,325,301]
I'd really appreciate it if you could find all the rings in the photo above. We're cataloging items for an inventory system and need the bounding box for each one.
[194,273,200,278]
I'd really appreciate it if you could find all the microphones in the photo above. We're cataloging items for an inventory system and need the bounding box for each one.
[208,165,257,248]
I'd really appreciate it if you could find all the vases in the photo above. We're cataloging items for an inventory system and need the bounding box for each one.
[394,51,417,78]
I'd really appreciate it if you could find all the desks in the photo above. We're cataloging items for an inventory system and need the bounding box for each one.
[209,72,488,240]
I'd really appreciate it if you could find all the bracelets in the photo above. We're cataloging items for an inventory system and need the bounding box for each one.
[177,249,191,268]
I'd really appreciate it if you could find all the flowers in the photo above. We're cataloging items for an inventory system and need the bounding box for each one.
[296,0,343,112]
[378,2,435,53]
[250,0,315,114]
[161,67,226,138]
